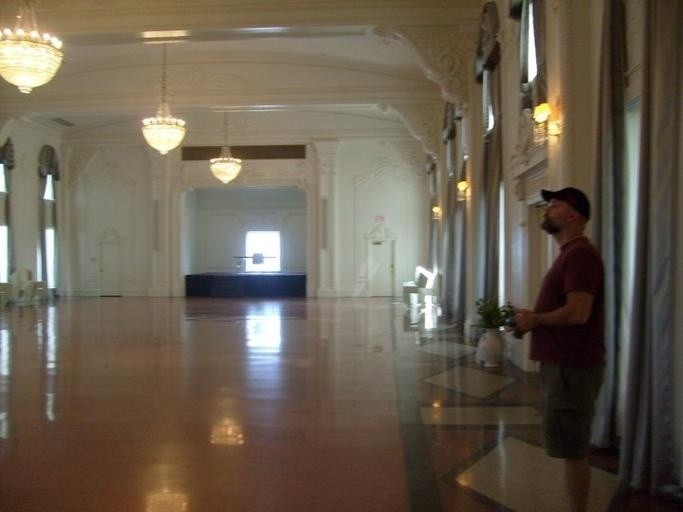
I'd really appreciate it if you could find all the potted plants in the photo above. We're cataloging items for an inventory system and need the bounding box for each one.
[471,297,520,369]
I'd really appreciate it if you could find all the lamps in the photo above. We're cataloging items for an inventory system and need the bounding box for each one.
[0,1,65,94]
[209,111,243,186]
[142,40,187,157]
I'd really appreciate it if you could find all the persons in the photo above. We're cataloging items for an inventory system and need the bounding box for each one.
[505,188,607,512]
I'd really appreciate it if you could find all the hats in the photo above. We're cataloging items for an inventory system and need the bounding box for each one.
[539,186,591,222]
